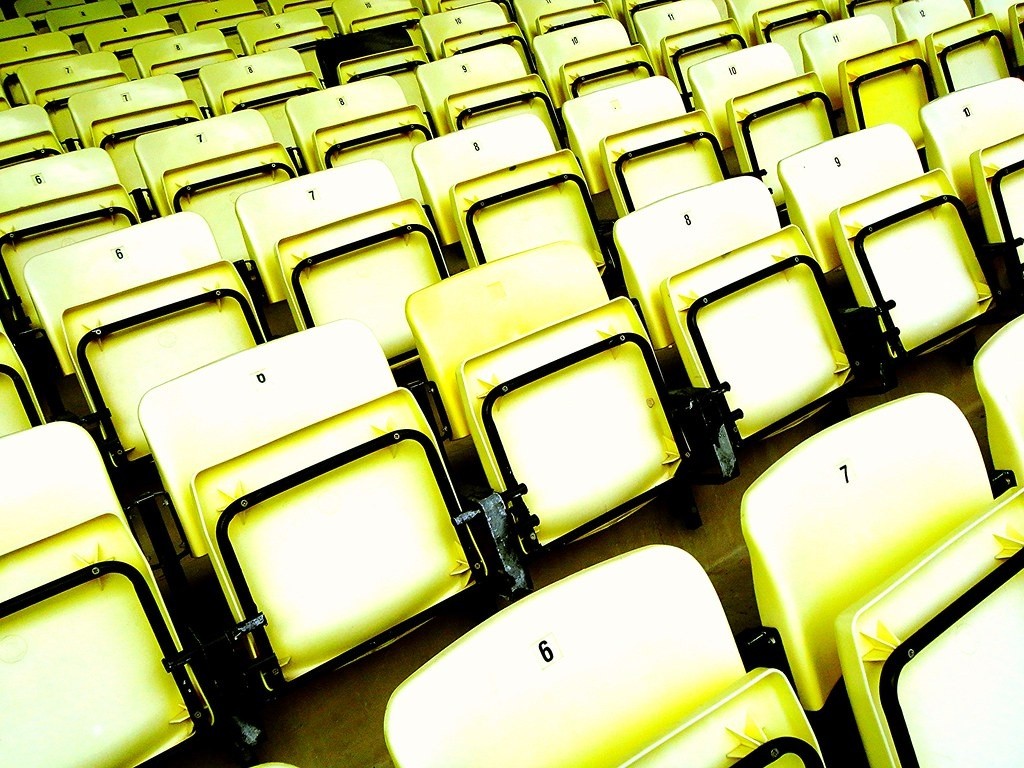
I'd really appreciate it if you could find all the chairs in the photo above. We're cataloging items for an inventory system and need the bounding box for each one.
[0,0,1024,768]
